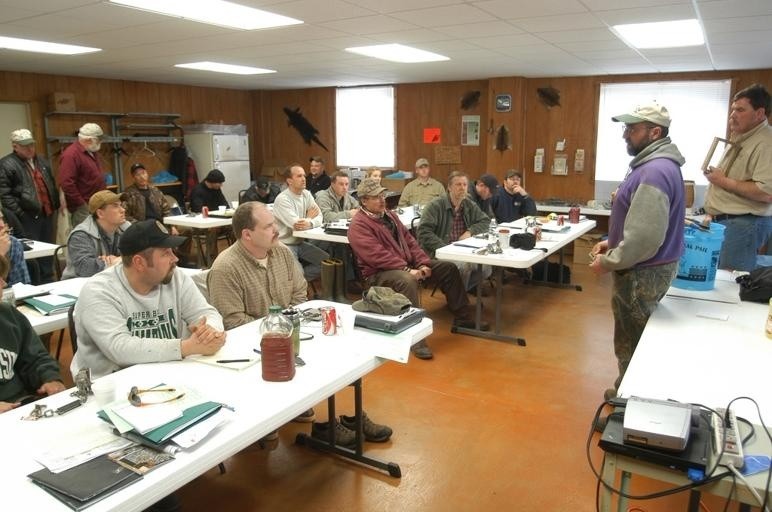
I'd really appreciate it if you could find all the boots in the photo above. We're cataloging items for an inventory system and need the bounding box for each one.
[451,305,490,333]
[411,339,433,358]
[330,258,352,304]
[319,259,335,302]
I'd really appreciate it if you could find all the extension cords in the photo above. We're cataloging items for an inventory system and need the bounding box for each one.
[712,408,745,469]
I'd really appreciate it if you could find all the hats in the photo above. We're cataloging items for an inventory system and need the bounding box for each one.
[612,100,671,128]
[207,169,224,183]
[309,156,325,162]
[505,169,522,178]
[11,129,37,146]
[357,177,389,198]
[79,122,104,136]
[415,159,429,167]
[352,285,412,316]
[479,175,500,196]
[88,189,129,214]
[130,163,146,173]
[118,218,188,256]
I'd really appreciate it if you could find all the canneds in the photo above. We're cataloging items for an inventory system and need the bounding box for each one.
[202,205,208,218]
[533,225,541,241]
[557,215,564,226]
[321,305,337,335]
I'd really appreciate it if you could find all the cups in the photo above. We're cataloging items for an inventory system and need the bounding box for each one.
[282,309,300,356]
[218,205,227,214]
[499,229,509,249]
[232,201,239,209]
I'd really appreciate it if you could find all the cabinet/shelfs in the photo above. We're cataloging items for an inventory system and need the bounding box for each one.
[44,110,121,194]
[115,111,183,189]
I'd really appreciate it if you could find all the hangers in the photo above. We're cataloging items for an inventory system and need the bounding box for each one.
[49,139,177,159]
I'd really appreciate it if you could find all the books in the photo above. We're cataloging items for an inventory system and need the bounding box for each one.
[26,376,235,512]
[556,213,586,222]
[188,341,260,372]
[11,276,96,314]
[536,221,571,233]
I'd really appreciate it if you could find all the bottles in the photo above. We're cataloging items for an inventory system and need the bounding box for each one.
[765,297,772,341]
[259,304,296,383]
[488,218,497,244]
[569,207,580,223]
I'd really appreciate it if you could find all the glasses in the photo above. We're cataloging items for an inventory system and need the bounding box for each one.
[361,290,384,315]
[128,387,185,407]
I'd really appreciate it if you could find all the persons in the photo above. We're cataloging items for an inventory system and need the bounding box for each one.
[59,123,105,229]
[0,128,60,285]
[189,168,236,269]
[347,176,492,362]
[207,201,315,440]
[305,158,333,194]
[126,163,198,270]
[417,168,539,300]
[242,179,281,204]
[69,218,226,382]
[314,171,368,296]
[0,253,68,413]
[365,165,383,183]
[701,83,772,274]
[398,161,447,207]
[589,101,686,400]
[60,189,132,281]
[272,163,331,300]
[0,207,33,288]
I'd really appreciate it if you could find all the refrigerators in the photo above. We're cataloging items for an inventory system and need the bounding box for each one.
[183,132,251,208]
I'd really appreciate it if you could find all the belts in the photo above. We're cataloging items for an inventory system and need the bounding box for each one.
[711,213,751,223]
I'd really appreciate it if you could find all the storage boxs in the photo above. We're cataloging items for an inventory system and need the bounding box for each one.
[46,91,76,113]
[572,238,602,265]
[682,180,695,209]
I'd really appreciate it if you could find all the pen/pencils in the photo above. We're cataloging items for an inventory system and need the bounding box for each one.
[217,359,249,363]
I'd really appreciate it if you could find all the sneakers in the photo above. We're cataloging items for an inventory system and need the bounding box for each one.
[311,420,365,449]
[339,412,392,442]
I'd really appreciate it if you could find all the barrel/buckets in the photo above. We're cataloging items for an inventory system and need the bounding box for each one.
[671,222,726,289]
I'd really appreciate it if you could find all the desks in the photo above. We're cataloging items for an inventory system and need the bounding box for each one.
[433,214,598,346]
[600,270,772,510]
[534,204,612,219]
[0,300,434,512]
